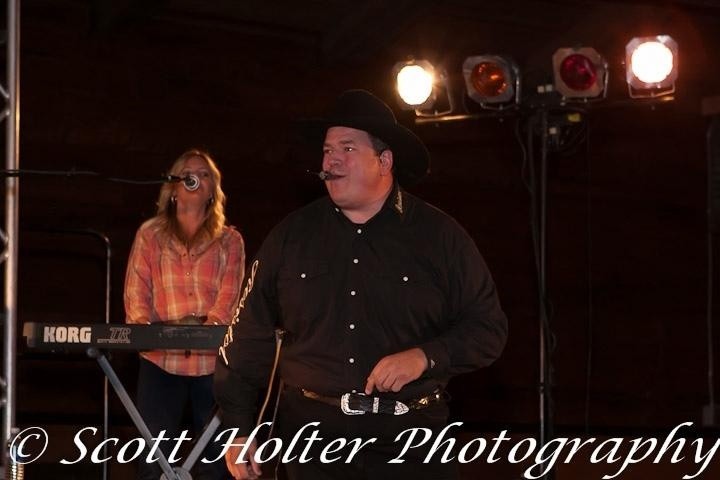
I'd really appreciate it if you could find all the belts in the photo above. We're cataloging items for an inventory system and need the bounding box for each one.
[277,376,446,417]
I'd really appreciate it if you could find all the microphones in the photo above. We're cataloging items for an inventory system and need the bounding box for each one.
[319,170,330,180]
[181,173,200,191]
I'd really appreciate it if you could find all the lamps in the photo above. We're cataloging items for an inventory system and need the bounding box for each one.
[380,29,678,124]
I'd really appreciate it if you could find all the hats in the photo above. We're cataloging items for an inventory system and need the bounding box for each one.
[296,88,432,177]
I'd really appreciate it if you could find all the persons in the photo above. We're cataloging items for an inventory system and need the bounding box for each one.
[121,148,246,480]
[213,87,508,480]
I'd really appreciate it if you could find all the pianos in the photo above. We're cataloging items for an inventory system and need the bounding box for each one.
[23,323,230,353]
